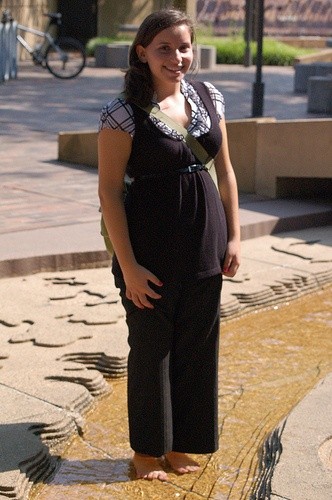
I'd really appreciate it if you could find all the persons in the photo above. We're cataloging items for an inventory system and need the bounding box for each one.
[97,8,243,482]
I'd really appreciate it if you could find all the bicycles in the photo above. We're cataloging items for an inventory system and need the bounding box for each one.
[1,10,86,80]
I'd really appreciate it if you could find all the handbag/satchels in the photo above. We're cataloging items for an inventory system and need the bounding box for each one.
[98,180,132,256]
